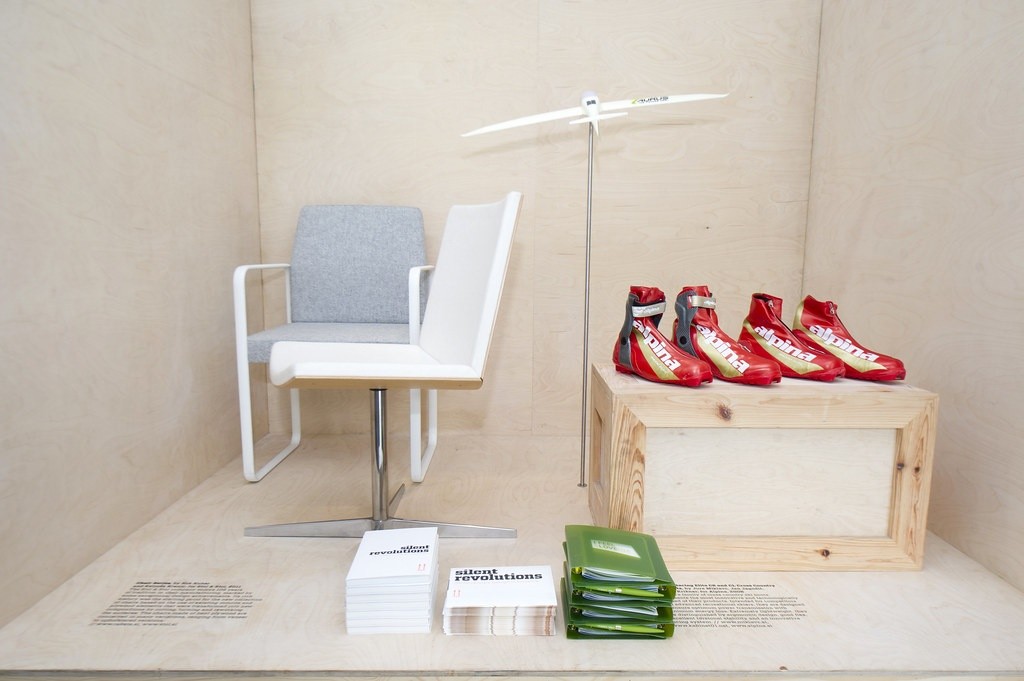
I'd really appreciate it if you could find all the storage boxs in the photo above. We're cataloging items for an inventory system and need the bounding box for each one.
[589,362,939,573]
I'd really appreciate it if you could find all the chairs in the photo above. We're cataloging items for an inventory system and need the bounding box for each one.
[243,192,523,543]
[232,204,437,483]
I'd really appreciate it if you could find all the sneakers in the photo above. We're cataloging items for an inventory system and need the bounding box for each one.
[612,285,909,389]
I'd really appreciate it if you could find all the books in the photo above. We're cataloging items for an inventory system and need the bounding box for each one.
[345,526,439,633]
[442,564,558,636]
